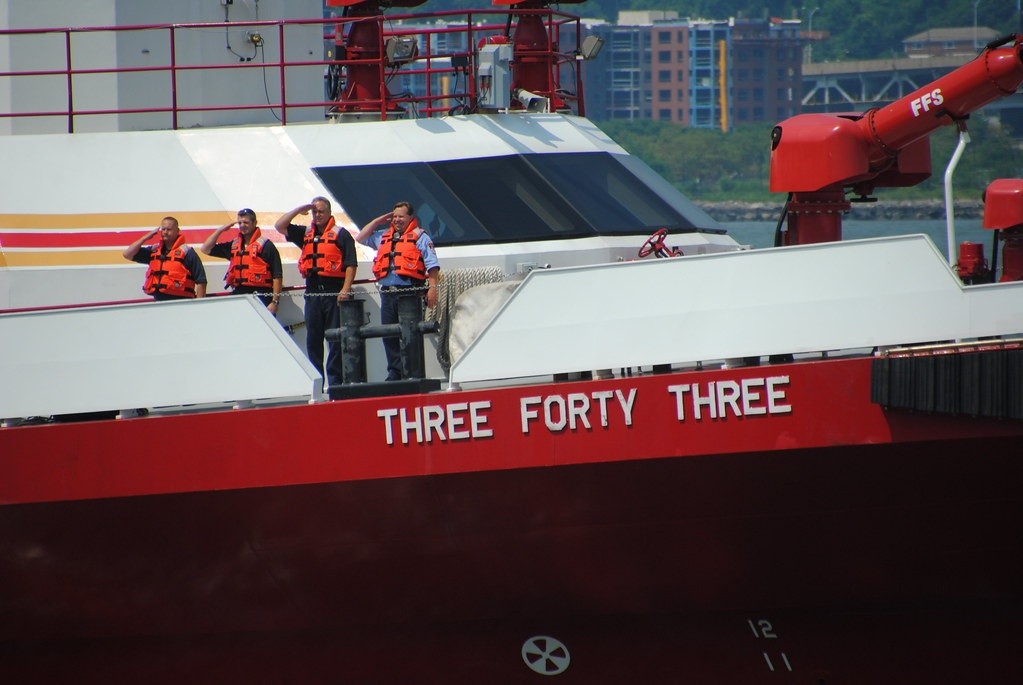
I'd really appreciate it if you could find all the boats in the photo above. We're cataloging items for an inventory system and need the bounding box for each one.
[0,0,1023,685]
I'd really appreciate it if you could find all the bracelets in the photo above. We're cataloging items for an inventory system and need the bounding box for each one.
[271,299,279,305]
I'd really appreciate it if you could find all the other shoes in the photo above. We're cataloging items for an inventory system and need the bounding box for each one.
[385,369,402,381]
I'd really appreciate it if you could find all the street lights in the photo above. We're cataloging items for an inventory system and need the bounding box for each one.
[973,2,981,52]
[808,7,821,65]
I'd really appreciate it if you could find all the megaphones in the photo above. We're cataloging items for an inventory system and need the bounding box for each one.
[513,89,548,113]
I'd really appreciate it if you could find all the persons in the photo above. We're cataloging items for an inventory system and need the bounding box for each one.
[274,197,357,393]
[355,202,440,382]
[122,216,206,301]
[200,208,282,318]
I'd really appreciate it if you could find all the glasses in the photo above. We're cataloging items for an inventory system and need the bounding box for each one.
[238,208,252,214]
